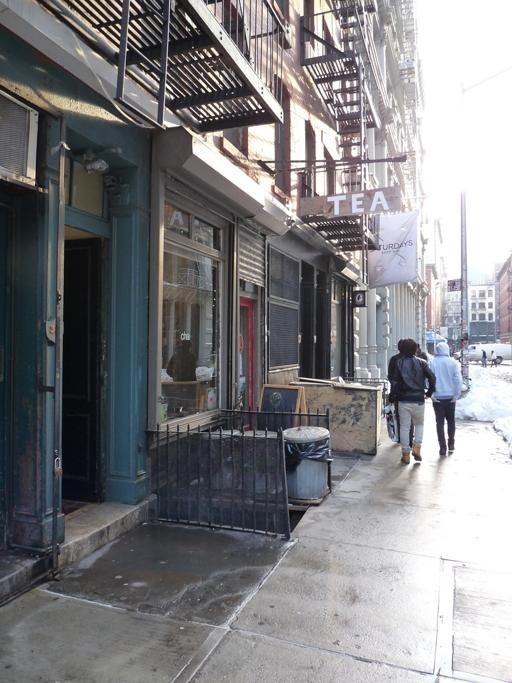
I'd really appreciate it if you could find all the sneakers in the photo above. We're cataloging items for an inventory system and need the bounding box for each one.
[439,449,454,455]
[401,453,410,463]
[412,447,421,460]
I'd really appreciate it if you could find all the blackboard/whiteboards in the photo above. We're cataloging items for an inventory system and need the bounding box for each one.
[258,384,303,427]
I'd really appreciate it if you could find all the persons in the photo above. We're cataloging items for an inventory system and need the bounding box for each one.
[388,337,436,464]
[489,349,497,367]
[414,342,429,390]
[167,338,197,417]
[424,341,463,457]
[388,337,415,447]
[480,348,486,367]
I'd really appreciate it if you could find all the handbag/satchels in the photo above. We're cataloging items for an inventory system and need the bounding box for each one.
[384,402,399,442]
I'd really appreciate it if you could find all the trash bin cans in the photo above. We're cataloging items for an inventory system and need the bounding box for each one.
[283,426,330,499]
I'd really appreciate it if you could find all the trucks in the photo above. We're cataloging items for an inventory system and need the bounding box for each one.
[453,344,512,364]
